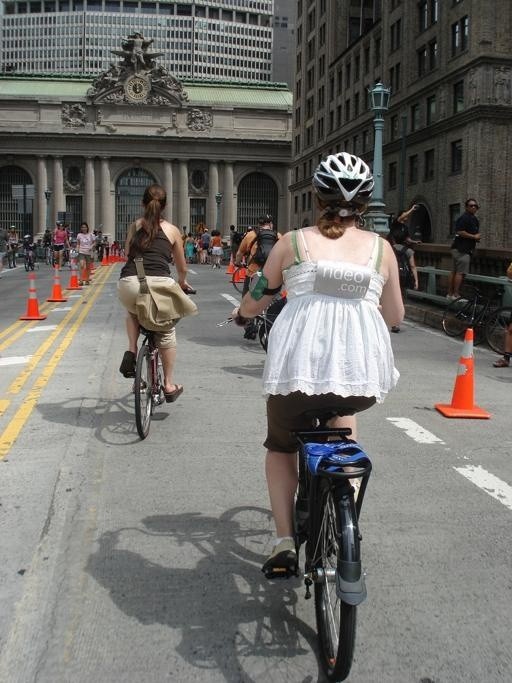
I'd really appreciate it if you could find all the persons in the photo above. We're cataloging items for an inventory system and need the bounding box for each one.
[4,221,121,283]
[447,199,481,302]
[119,184,197,402]
[122,32,149,75]
[388,231,418,333]
[493,262,511,368]
[391,204,422,247]
[232,152,405,572]
[234,215,283,338]
[183,225,252,269]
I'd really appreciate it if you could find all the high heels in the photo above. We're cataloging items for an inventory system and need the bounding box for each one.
[164,383,184,403]
[120,350,136,374]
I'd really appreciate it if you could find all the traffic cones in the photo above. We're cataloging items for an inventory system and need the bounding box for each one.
[46,264,66,301]
[225,251,235,273]
[239,255,247,279]
[65,258,82,289]
[90,263,95,273]
[81,258,88,285]
[432,345,492,418]
[230,265,240,282]
[100,247,127,265]
[19,274,46,319]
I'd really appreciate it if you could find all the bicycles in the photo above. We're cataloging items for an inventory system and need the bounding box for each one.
[232,262,284,339]
[217,311,401,681]
[126,287,197,439]
[6,241,17,267]
[485,306,512,356]
[19,246,34,271]
[442,277,505,346]
[44,244,53,264]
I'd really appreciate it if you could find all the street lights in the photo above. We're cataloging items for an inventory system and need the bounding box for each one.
[359,80,393,238]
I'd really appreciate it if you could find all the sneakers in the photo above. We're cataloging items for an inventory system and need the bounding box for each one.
[493,356,508,367]
[447,293,469,302]
[353,478,361,501]
[390,325,399,331]
[243,330,256,340]
[261,539,296,570]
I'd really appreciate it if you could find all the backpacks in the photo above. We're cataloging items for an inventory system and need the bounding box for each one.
[252,227,278,266]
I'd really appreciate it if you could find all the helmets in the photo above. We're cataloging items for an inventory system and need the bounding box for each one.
[313,153,374,207]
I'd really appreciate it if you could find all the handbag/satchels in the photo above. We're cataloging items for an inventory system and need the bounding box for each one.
[138,281,197,334]
